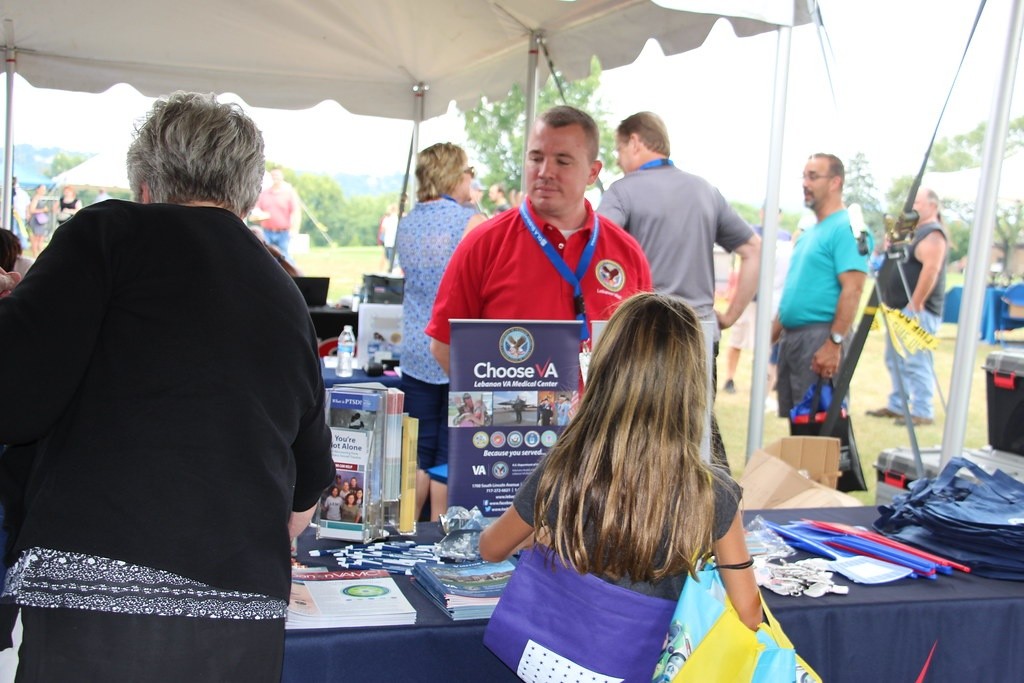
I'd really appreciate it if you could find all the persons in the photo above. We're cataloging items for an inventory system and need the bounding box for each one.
[322,474,364,522]
[10,177,112,257]
[392,144,489,525]
[377,183,512,272]
[427,101,652,406]
[725,151,949,435]
[480,293,760,682]
[453,391,571,428]
[0,228,22,299]
[592,111,761,475]
[246,166,301,278]
[0,92,341,683]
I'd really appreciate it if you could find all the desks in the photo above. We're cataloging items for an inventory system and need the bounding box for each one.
[282,507,1024,683]
[308,306,358,341]
[320,358,401,388]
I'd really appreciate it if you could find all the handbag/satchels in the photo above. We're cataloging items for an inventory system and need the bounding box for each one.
[35,213,50,225]
[873,456,1024,584]
[791,373,869,493]
[651,465,825,683]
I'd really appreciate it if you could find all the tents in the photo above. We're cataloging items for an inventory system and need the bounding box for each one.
[0,0,949,483]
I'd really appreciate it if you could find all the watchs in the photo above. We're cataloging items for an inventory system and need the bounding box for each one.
[277,254,286,263]
[830,332,844,345]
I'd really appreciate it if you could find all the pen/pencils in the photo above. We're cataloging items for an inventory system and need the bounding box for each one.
[308,548,342,557]
[334,540,445,575]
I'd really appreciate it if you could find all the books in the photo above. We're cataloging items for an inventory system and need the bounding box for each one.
[283,567,417,630]
[412,555,516,621]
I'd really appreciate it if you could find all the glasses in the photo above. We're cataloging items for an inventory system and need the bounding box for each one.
[464,166,476,179]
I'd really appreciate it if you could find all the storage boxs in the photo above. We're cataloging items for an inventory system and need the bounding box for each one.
[872,348,1024,506]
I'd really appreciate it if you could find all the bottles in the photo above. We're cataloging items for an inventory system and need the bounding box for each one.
[335,324,355,378]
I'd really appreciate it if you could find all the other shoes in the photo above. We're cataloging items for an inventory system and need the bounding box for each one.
[865,407,906,419]
[895,414,934,427]
